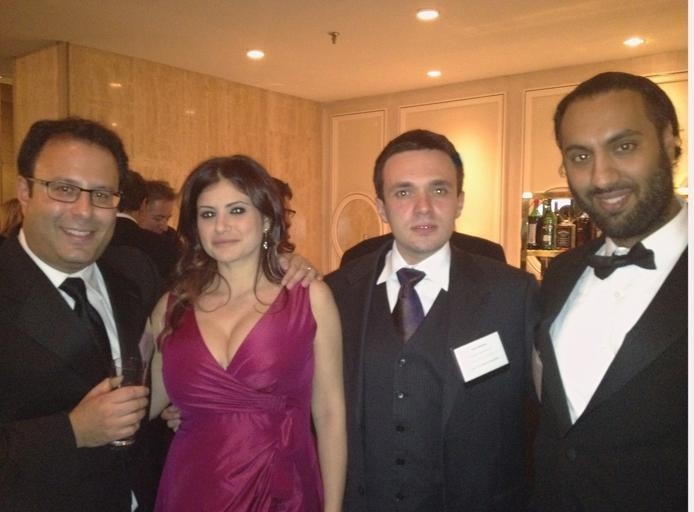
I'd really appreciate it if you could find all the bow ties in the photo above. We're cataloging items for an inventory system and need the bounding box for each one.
[584,242,655,279]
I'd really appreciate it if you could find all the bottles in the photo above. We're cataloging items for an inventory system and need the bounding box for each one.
[525,196,577,252]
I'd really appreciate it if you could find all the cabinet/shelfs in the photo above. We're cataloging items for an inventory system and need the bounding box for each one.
[518,189,596,280]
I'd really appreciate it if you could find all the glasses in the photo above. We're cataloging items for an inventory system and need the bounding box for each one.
[27,177,122,210]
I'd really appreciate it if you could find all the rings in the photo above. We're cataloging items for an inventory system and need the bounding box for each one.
[305,266,312,271]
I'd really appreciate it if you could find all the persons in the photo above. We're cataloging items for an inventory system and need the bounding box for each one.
[541,73,688,512]
[269,178,296,232]
[338,231,507,270]
[113,171,182,281]
[136,156,349,512]
[0,198,23,243]
[137,181,184,251]
[0,117,324,512]
[311,130,544,512]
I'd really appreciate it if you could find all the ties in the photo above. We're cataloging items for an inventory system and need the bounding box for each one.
[392,268,424,342]
[61,278,111,365]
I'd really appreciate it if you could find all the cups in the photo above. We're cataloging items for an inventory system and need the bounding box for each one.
[575,217,598,247]
[104,357,150,447]
